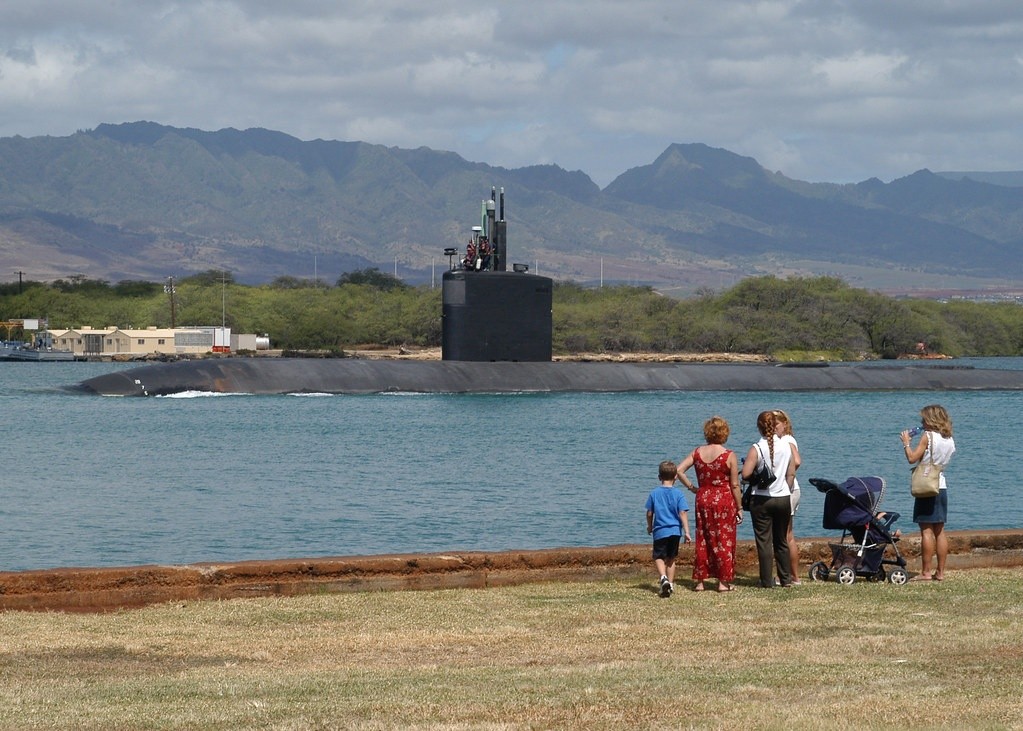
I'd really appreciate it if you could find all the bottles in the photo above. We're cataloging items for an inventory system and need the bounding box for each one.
[909,427,923,437]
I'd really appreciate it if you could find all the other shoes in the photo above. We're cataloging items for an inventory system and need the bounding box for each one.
[756,575,801,588]
[669,583,673,593]
[660,578,671,597]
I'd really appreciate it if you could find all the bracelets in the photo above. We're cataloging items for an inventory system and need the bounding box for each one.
[903,445,910,449]
[738,506,743,510]
[688,484,694,490]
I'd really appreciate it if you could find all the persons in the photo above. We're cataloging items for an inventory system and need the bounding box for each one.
[481,238,496,272]
[900,405,956,581]
[464,244,476,270]
[676,414,744,592]
[645,461,692,598]
[741,409,802,588]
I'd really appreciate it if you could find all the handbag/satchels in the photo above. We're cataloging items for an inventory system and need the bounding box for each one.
[910,464,942,497]
[754,442,777,489]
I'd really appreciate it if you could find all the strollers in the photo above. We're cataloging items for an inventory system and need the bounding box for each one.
[808,474,910,587]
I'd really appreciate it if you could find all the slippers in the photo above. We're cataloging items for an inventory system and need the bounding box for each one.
[694,584,709,592]
[909,575,932,582]
[718,585,734,592]
[932,575,943,581]
